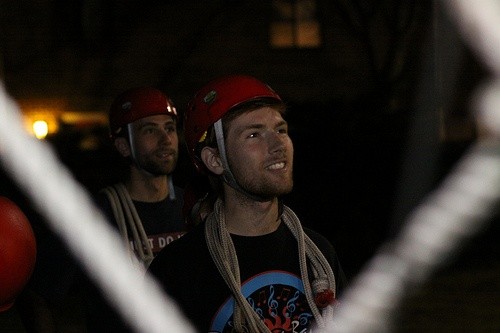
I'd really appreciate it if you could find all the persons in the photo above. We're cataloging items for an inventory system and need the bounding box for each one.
[145,75,345,333]
[103,86,210,275]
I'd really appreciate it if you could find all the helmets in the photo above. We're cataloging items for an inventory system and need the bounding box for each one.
[0,196,36,315]
[107,86,180,140]
[181,71,283,176]
